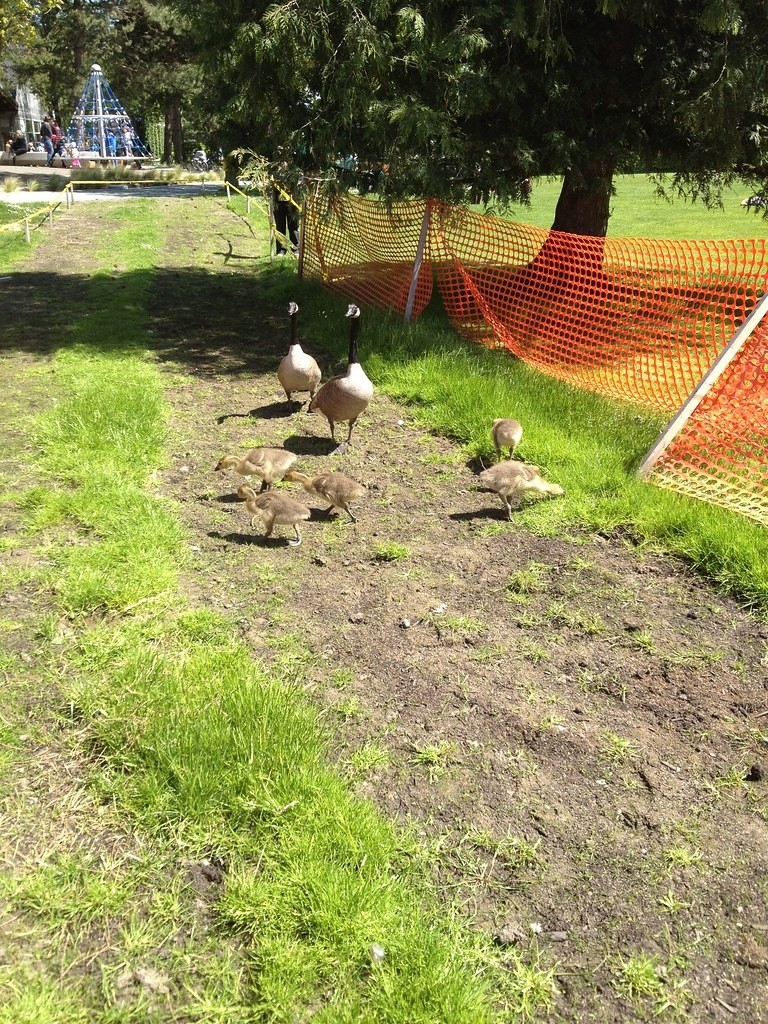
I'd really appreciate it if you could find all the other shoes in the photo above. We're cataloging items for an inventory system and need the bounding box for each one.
[277,250,283,256]
[294,251,299,255]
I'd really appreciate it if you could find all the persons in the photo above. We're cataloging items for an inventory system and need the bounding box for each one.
[41,115,135,170]
[330,151,533,206]
[10,129,28,166]
[267,160,300,256]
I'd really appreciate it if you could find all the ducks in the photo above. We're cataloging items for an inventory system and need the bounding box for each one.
[491,418,523,463]
[214,447,366,547]
[480,459,563,522]
[278,302,374,445]
